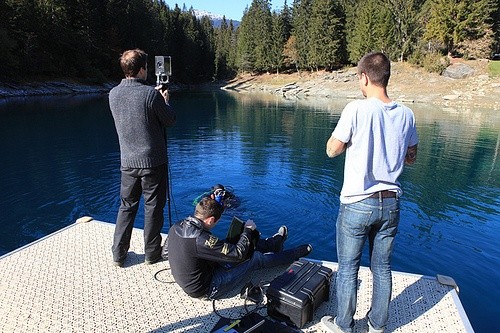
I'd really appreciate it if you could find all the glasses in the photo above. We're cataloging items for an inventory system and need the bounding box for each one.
[214,190,226,196]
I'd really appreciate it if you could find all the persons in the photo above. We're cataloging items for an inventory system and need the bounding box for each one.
[109,50,164,265]
[326,53,418,333]
[193,184,234,206]
[161,198,313,299]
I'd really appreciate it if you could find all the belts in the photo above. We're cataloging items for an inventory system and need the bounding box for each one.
[369,190,396,198]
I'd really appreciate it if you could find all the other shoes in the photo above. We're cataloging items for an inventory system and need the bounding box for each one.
[145,246,164,264]
[114,253,128,266]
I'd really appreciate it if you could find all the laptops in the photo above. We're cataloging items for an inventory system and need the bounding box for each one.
[213,319,265,333]
[217,216,261,271]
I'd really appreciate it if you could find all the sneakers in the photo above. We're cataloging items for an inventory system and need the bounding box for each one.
[284,244,312,260]
[321,315,352,333]
[366,314,384,333]
[269,224,287,253]
